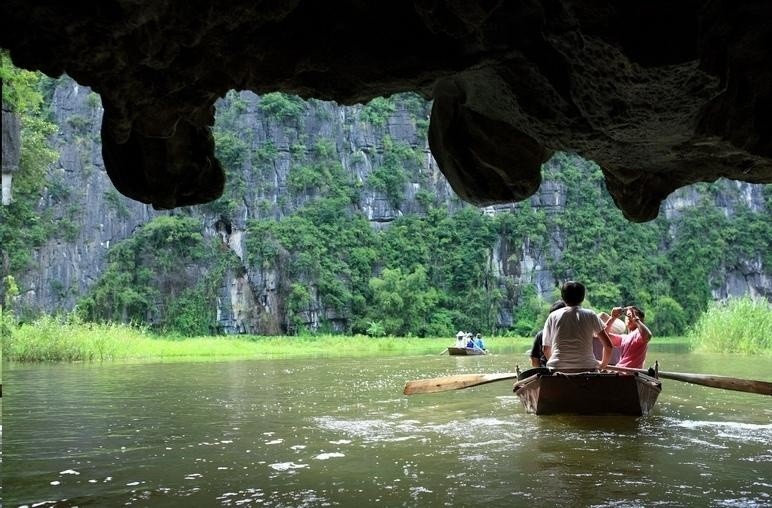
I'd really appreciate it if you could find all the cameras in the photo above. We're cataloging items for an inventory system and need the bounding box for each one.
[620,309,627,314]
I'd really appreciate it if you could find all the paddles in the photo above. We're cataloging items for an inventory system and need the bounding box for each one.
[403,372,516,395]
[605,365,772,396]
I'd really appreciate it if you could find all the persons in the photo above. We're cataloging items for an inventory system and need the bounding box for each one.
[455,331,486,349]
[530,280,652,375]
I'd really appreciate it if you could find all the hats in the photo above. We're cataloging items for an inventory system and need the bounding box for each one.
[592,312,626,338]
[456,331,466,337]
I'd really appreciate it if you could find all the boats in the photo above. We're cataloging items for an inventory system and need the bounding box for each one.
[447,346,489,355]
[512,360,663,416]
